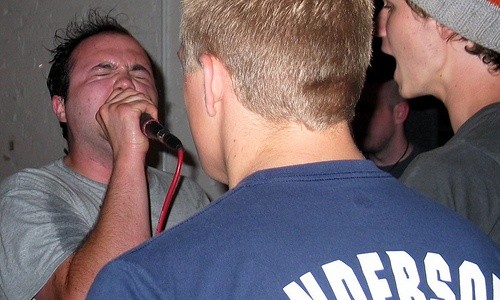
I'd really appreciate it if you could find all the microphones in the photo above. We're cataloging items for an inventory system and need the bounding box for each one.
[139,113,182,151]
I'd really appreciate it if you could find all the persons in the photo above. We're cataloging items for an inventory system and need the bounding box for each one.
[85,0,500,300]
[351,59,426,180]
[374,0,500,255]
[0,7,210,300]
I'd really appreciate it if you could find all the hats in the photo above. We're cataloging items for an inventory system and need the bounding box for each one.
[412,0,500,54]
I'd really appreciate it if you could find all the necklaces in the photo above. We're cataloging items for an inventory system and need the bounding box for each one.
[388,142,409,173]
